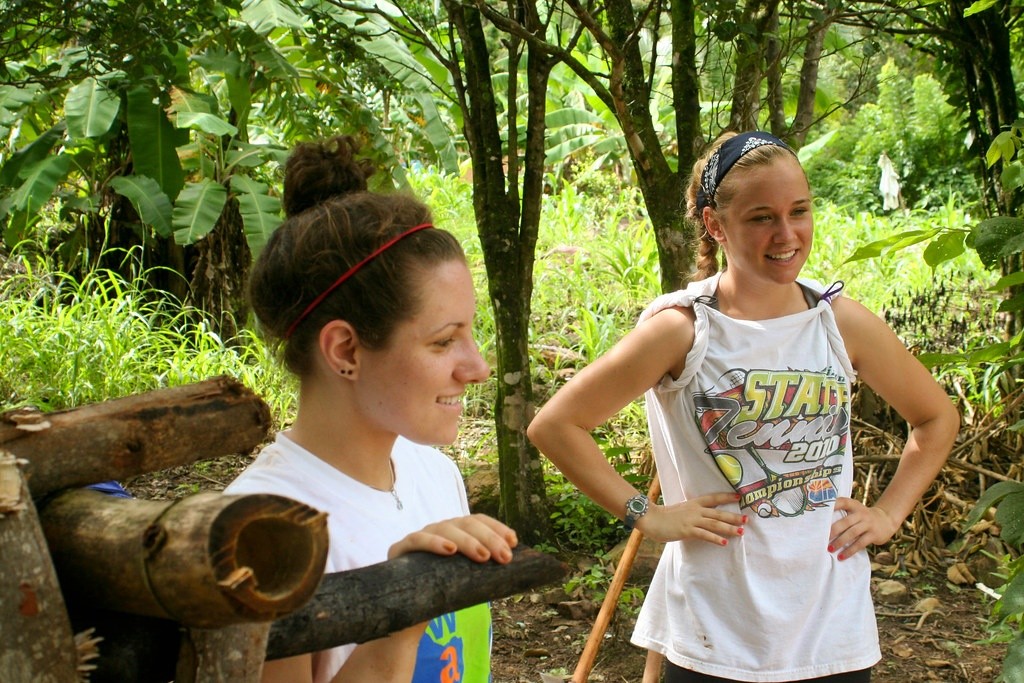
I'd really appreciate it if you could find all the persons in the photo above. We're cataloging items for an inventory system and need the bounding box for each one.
[523,132,960,683]
[220,137,519,683]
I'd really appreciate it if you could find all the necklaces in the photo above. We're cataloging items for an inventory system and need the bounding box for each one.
[371,458,402,511]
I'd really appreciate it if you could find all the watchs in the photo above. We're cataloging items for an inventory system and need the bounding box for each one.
[624,494,649,528]
